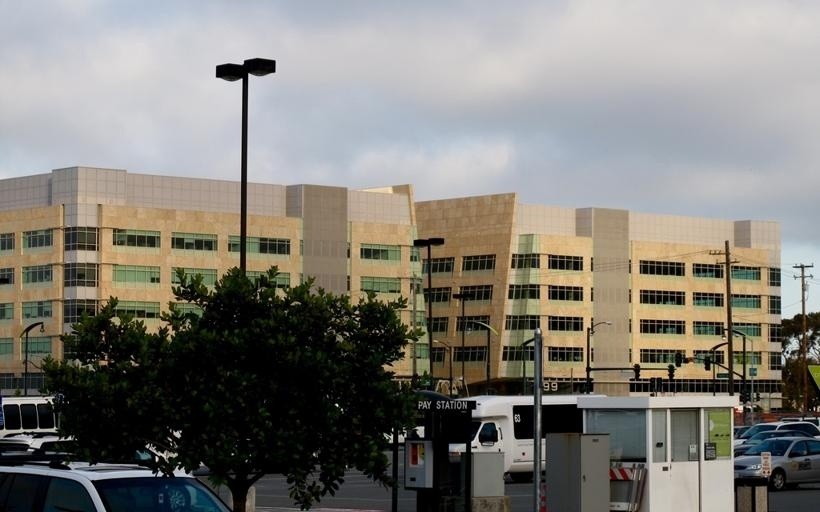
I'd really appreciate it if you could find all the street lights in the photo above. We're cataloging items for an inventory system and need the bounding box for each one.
[722,328,746,423]
[453,293,475,397]
[586,322,612,393]
[433,339,452,398]
[216,59,275,273]
[25,322,44,396]
[414,238,444,391]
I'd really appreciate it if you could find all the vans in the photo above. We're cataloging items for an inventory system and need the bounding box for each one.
[0,396,78,450]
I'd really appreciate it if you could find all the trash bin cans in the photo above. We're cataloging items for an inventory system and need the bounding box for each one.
[735,475,769,512]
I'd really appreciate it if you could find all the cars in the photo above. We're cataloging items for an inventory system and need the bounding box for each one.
[0,460,233,512]
[734,417,820,490]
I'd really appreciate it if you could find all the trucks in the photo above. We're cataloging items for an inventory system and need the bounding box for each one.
[449,393,607,483]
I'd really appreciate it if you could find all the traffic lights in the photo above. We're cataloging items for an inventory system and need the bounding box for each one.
[675,353,682,367]
[668,365,674,378]
